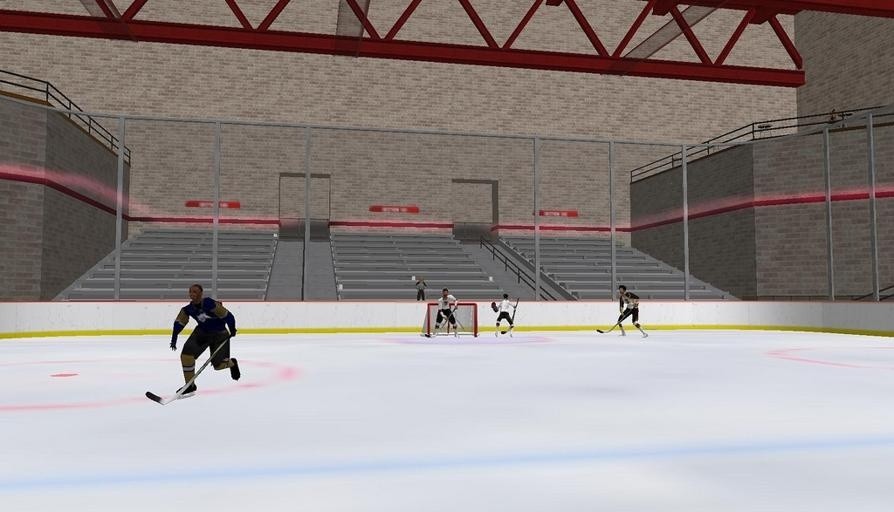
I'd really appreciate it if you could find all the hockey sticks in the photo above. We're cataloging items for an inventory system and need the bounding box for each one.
[146,335,231,405]
[501,298,519,334]
[425,308,455,337]
[597,307,635,333]
